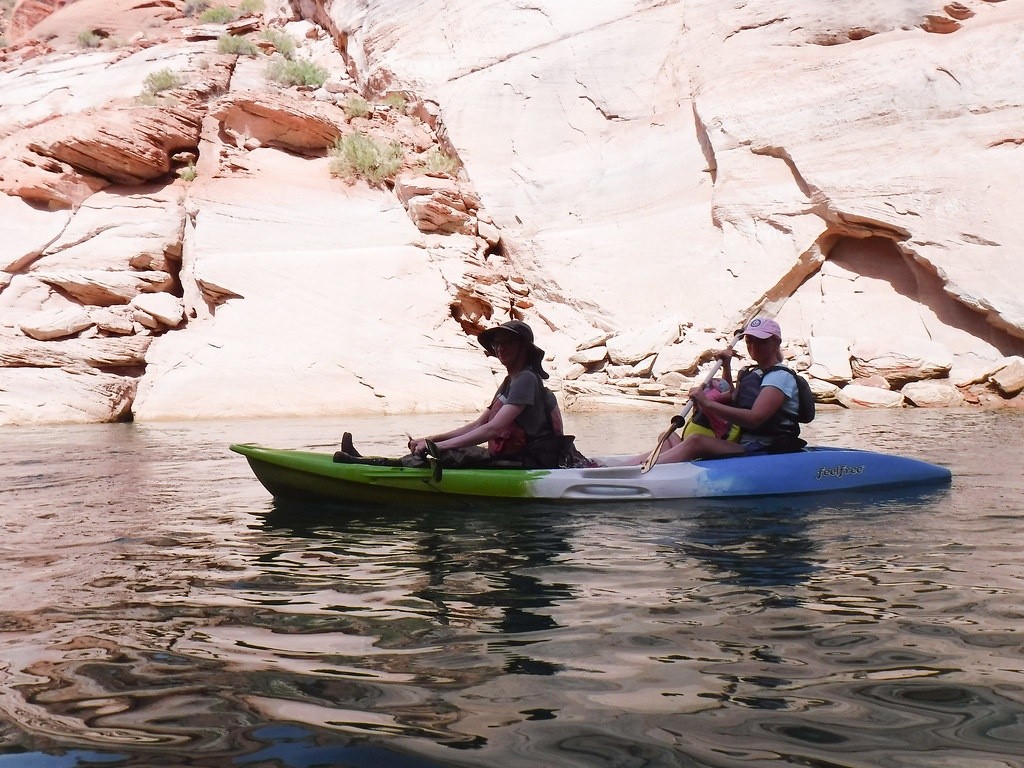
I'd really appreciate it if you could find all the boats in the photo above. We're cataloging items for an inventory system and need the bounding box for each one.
[228,442,955,511]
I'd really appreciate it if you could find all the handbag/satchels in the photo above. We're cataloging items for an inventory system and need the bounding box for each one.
[526,435,591,469]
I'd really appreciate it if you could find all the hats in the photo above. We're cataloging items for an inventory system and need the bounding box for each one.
[740,317,782,339]
[477,321,550,380]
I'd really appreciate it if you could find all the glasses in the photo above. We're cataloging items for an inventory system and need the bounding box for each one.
[489,337,520,349]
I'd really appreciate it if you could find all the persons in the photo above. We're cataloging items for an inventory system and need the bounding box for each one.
[333,320,563,470]
[610,316,798,467]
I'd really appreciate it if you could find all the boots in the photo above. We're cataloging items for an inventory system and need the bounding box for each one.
[333,451,389,466]
[341,432,362,457]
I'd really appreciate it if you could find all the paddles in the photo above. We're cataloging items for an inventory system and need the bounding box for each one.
[405,431,443,484]
[638,302,767,476]
